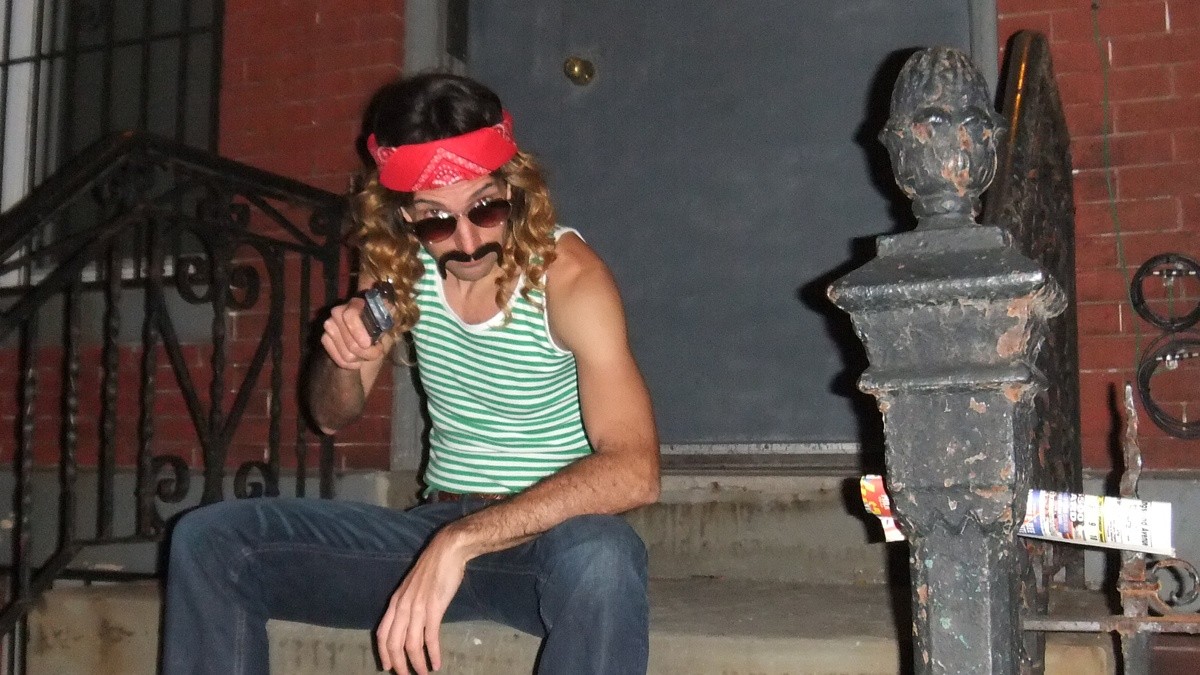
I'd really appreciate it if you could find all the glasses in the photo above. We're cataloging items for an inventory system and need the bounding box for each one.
[401,185,518,242]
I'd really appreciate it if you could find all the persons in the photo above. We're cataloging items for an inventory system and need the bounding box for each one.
[164,68,661,675]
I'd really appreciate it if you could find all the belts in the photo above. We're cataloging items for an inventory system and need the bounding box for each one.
[436,491,511,503]
